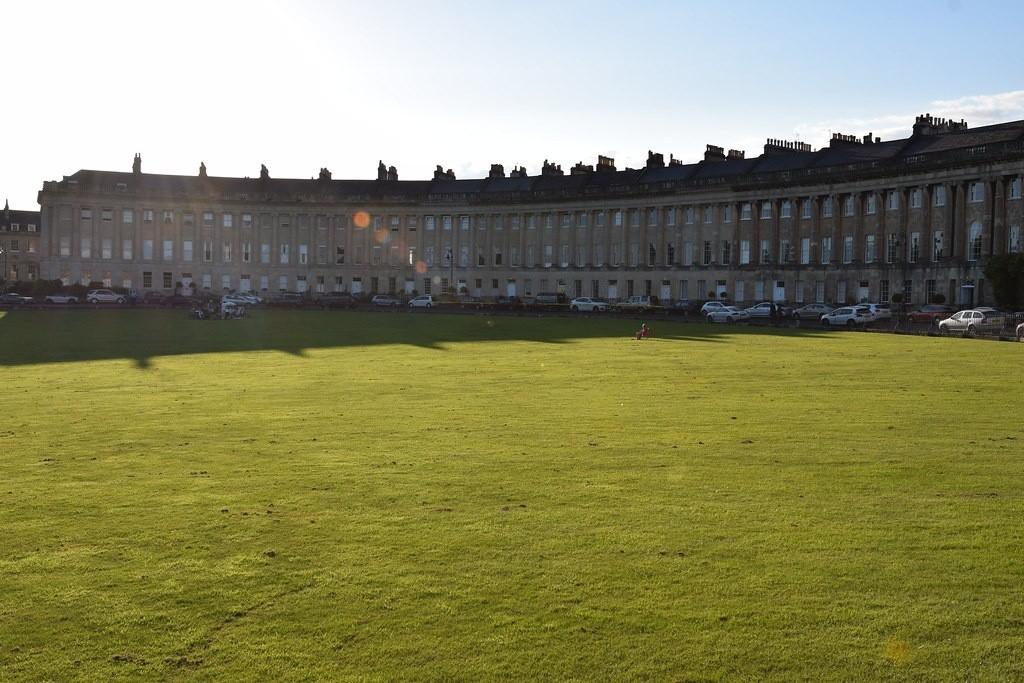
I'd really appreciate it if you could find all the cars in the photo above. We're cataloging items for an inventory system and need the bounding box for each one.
[43,292,78,304]
[0,291,33,305]
[745,301,791,318]
[570,298,610,312]
[664,299,699,317]
[905,304,959,325]
[821,305,875,330]
[705,306,747,325]
[792,303,835,320]
[701,302,741,315]
[219,295,248,306]
[234,293,263,305]
[160,295,199,308]
[144,291,166,304]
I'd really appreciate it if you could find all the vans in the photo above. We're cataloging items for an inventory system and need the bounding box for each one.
[408,295,437,307]
[323,292,350,308]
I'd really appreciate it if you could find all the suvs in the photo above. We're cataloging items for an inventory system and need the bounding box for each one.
[86,289,125,304]
[371,294,404,307]
[938,307,1005,337]
[857,303,892,322]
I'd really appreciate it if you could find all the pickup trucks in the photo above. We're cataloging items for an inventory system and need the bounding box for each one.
[616,296,663,314]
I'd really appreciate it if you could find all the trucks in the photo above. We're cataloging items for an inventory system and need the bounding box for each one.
[271,293,304,308]
[535,292,557,303]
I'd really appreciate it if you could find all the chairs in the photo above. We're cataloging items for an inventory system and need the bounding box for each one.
[638,327,650,339]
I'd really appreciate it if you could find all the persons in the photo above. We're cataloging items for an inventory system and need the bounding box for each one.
[769,301,787,328]
